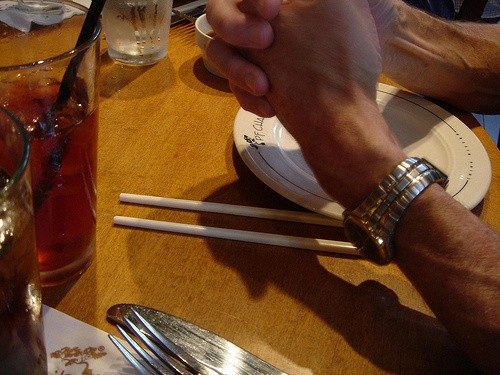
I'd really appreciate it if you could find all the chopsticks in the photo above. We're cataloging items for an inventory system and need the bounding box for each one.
[113,192,367,256]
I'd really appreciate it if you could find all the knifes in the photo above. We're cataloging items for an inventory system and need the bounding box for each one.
[106,304,288,375]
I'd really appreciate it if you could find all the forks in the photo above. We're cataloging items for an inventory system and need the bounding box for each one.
[108,307,204,375]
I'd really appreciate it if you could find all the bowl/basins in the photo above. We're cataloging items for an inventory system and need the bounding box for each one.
[195,12,240,80]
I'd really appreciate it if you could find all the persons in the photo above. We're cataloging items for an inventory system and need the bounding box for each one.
[204,0,500,375]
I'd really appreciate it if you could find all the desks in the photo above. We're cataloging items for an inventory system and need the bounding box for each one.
[0,0,500,375]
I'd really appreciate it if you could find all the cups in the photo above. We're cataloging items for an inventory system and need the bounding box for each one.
[0,107,47,375]
[102,0,173,66]
[0,0,102,287]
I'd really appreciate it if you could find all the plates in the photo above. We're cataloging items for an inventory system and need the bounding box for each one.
[232,83,492,220]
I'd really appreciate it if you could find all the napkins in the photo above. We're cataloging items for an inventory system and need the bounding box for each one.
[42,304,176,375]
[0,1,85,34]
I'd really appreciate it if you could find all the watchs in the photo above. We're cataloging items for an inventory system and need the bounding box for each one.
[341,157,449,266]
